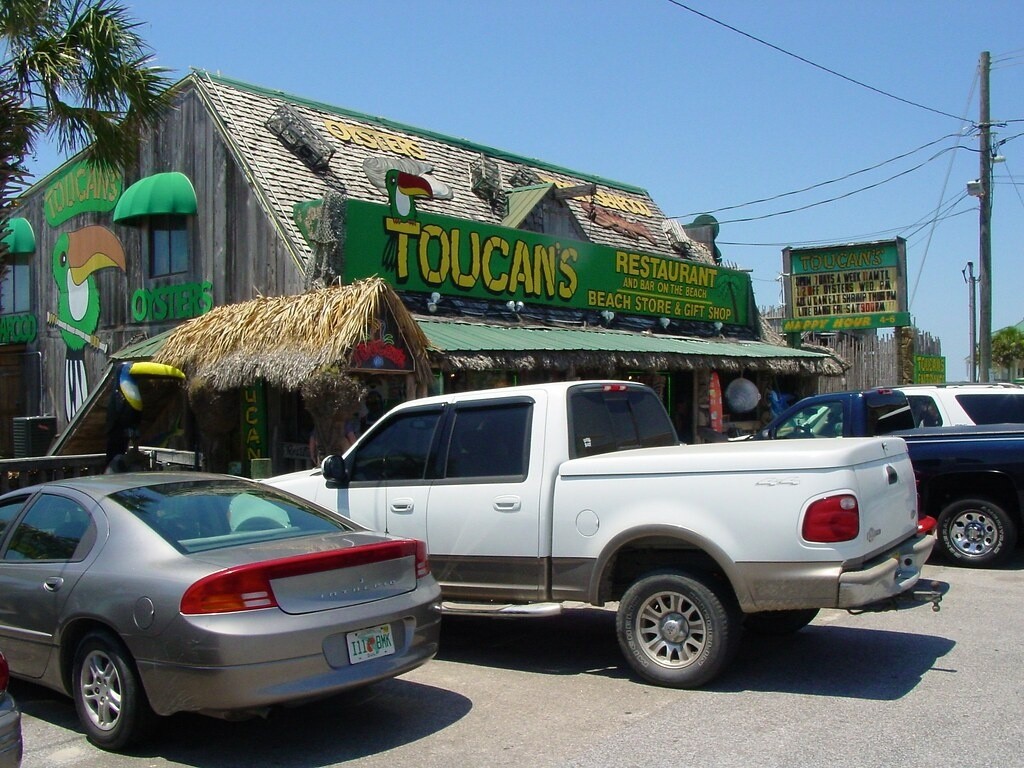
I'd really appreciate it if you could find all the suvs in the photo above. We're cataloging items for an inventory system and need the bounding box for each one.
[872,382,1024,428]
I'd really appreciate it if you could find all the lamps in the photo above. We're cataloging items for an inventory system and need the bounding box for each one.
[428,292,440,312]
[602,312,615,326]
[661,318,670,328]
[714,322,723,330]
[507,301,524,312]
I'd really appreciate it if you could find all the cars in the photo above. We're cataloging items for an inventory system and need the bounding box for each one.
[0,471,442,749]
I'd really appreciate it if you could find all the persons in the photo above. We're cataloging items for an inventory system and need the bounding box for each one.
[105,447,149,475]
[309,418,357,465]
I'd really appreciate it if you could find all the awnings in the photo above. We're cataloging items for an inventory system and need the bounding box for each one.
[408,306,850,378]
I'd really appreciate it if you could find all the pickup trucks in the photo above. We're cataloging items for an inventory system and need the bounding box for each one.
[257,380,937,686]
[748,388,1024,567]
